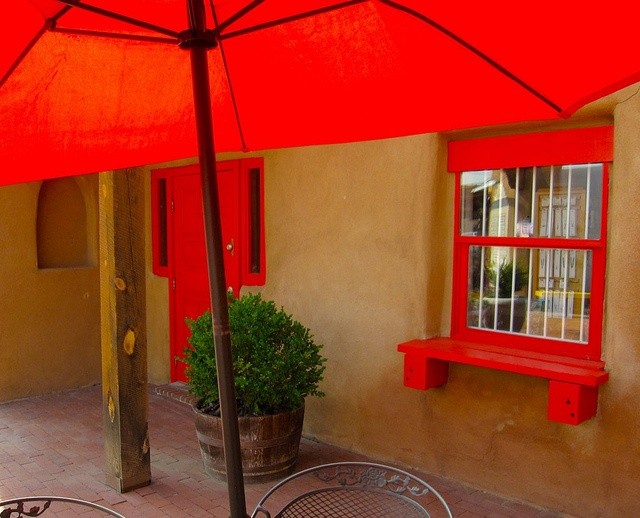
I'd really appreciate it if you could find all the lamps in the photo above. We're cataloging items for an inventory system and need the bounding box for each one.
[0,497,120,517]
[247,460,454,518]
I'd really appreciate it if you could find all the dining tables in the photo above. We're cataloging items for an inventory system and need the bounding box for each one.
[480,260,533,332]
[173,291,327,485]
